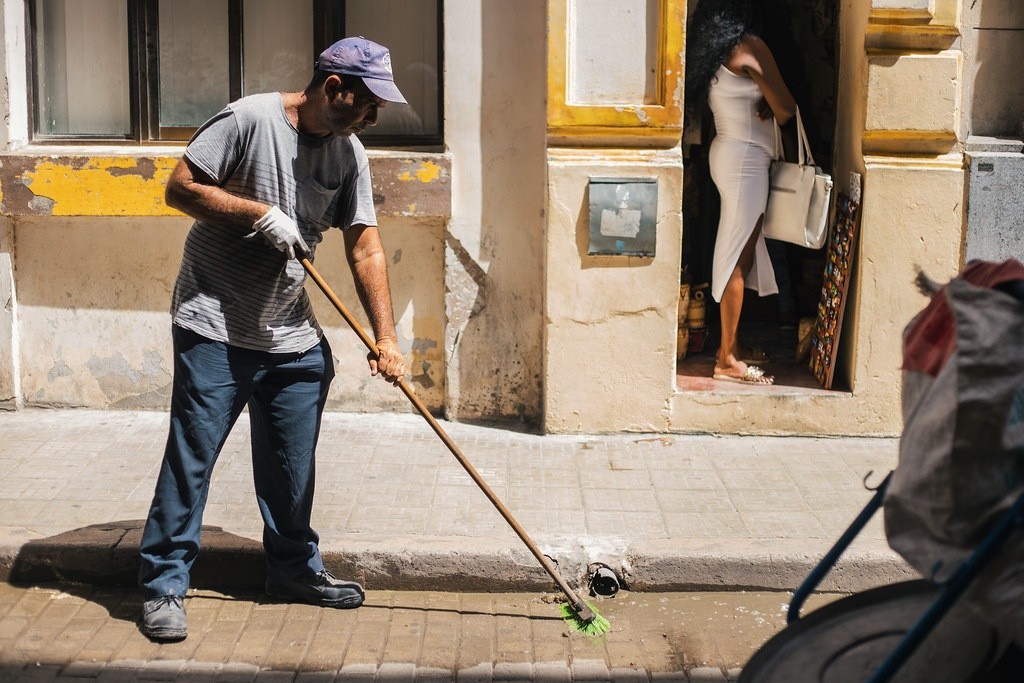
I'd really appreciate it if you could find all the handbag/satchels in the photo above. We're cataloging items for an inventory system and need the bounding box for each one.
[762,103,834,250]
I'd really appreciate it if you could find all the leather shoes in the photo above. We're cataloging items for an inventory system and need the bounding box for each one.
[142,595,189,642]
[265,570,365,610]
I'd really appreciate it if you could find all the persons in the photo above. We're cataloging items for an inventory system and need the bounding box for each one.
[685,0,797,386]
[138,35,408,643]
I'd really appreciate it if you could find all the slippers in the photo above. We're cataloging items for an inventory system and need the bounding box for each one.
[713,345,777,386]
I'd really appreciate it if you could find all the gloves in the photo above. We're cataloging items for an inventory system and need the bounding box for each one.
[252,206,309,260]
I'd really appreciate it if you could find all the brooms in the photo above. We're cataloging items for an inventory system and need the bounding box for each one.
[291,252,614,640]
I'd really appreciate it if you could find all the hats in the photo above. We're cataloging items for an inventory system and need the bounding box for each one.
[316,36,408,105]
